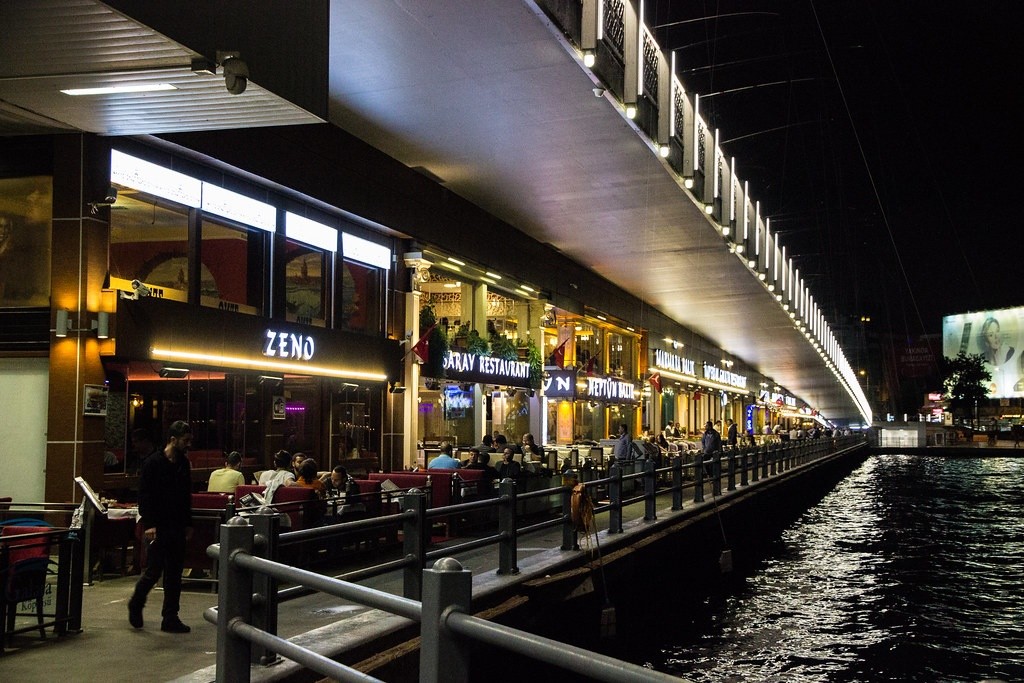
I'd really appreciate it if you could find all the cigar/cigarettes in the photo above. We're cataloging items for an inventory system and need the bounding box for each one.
[150,540,154,544]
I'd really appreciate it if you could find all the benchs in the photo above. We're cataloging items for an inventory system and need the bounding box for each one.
[460,452,522,476]
[195,457,257,471]
[369,473,432,545]
[424,469,491,528]
[391,471,461,538]
[235,486,318,567]
[348,480,382,551]
[545,440,702,508]
[135,494,228,593]
[187,450,224,469]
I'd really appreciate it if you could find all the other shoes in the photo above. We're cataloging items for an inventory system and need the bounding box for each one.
[128,598,145,628]
[162,616,191,633]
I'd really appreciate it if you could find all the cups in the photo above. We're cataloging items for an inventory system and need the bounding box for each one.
[340,493,346,500]
[526,453,531,462]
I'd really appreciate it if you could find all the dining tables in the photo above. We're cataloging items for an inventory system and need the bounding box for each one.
[64,501,147,582]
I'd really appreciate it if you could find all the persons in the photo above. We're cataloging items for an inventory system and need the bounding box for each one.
[127,420,192,634]
[976,317,1014,365]
[206,420,853,507]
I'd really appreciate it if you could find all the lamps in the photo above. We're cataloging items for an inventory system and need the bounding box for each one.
[91,312,109,339]
[55,309,72,337]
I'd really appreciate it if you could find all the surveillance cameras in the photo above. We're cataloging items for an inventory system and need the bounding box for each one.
[407,331,413,339]
[105,187,117,203]
[131,279,149,297]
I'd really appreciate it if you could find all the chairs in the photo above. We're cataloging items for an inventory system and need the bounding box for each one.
[0,526,55,653]
